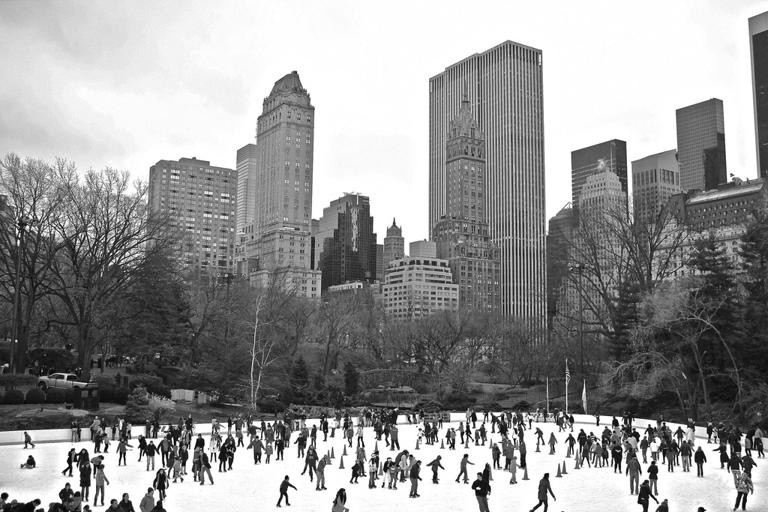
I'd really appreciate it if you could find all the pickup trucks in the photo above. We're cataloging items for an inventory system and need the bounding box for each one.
[37,372,98,395]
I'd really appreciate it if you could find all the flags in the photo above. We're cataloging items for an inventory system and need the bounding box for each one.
[565,364,570,384]
[582,386,587,413]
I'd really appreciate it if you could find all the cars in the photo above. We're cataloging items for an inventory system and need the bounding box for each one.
[91,352,132,364]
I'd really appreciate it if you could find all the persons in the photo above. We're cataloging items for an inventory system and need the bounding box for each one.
[2,363,9,391]
[0,408,766,512]
[28,366,82,377]
[90,355,124,369]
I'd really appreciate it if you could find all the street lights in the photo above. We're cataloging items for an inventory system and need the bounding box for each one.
[8,213,43,375]
[221,273,235,349]
[567,261,595,415]
